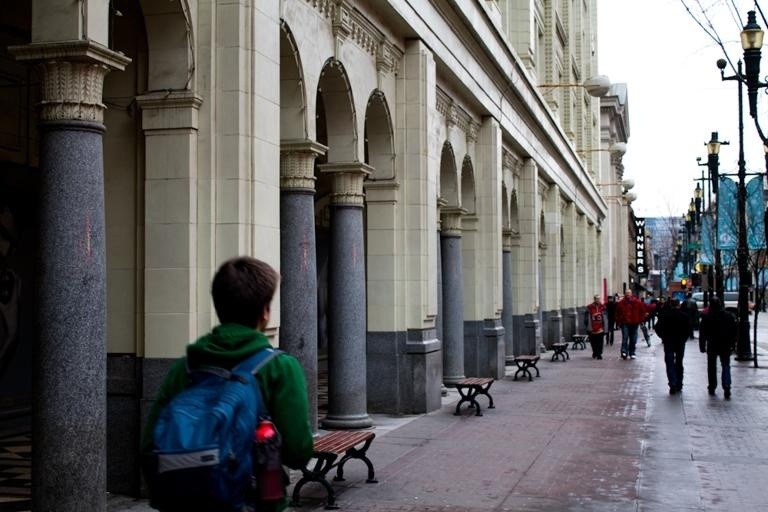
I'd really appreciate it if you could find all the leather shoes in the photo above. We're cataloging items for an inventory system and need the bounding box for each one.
[669,383,675,394]
[708,386,715,395]
[723,384,731,399]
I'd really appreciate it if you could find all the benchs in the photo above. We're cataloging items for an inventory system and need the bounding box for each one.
[571,332,586,352]
[447,373,499,417]
[511,352,541,382]
[288,427,379,510]
[550,341,571,363]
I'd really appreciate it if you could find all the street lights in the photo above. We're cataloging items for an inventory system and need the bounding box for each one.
[661,178,725,310]
[692,131,768,362]
[739,10,768,145]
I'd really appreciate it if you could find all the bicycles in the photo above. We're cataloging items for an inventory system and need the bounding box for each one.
[640,317,653,347]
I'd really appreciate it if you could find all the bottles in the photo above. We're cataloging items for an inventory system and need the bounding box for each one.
[256,413,286,502]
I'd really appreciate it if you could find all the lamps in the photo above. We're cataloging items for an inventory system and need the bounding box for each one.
[597,178,635,192]
[576,140,629,163]
[603,190,639,205]
[534,74,615,104]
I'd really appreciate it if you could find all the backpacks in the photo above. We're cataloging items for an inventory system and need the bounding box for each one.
[151,347,284,511]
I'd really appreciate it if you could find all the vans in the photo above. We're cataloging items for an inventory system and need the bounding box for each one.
[677,291,738,316]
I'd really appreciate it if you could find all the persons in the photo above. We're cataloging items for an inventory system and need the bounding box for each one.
[584,288,739,401]
[139,255,313,511]
